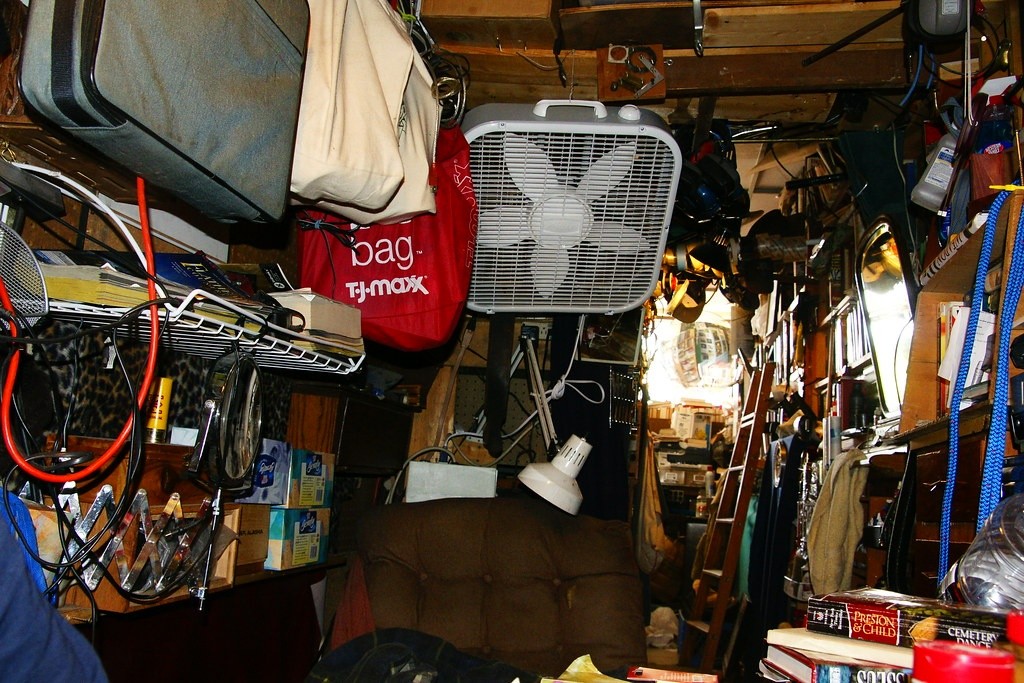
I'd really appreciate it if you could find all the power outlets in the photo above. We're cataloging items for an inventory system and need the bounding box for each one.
[522,320,551,340]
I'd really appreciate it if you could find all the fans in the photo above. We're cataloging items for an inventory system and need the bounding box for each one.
[459,97,681,317]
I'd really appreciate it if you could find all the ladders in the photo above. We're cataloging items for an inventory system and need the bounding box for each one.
[680,360,780,672]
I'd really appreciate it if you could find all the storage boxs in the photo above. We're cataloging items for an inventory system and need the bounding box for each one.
[233,437,335,572]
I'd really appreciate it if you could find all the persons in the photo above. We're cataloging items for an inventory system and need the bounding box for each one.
[0,515,108,683]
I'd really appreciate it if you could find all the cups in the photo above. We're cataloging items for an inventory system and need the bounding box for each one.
[861,412,873,427]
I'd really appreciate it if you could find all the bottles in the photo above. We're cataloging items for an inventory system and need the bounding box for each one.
[911,122,961,213]
[848,383,865,429]
[910,493,1024,683]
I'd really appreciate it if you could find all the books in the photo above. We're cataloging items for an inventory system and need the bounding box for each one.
[755,585,1013,683]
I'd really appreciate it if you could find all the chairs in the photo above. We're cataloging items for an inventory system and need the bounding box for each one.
[338,500,647,677]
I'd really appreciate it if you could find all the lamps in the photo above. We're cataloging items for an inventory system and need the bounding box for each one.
[453,334,593,516]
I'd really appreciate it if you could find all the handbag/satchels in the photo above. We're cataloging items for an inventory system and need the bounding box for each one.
[290,0,445,226]
[294,101,478,350]
[15,0,311,222]
